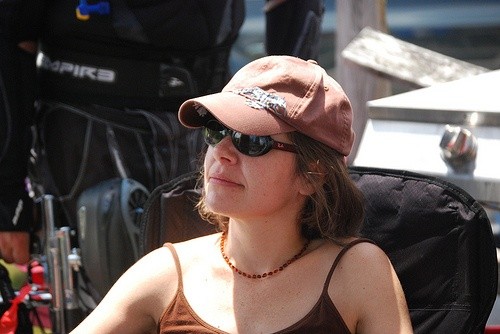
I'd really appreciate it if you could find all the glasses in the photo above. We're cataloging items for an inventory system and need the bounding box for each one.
[202,118,302,157]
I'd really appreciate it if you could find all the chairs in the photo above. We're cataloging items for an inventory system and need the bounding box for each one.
[139,166,500,334]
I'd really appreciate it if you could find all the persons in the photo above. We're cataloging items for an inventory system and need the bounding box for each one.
[68,56,414,334]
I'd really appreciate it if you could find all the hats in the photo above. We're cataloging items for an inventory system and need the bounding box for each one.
[178,54,355,158]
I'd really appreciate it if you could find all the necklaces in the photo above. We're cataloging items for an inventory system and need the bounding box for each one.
[220,228,316,279]
